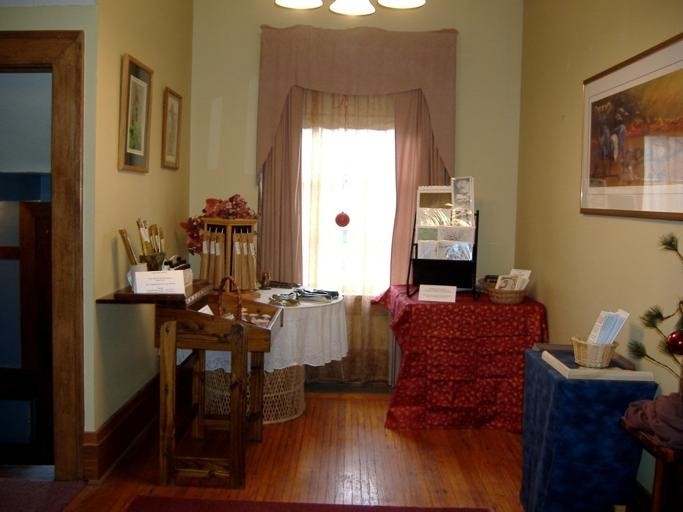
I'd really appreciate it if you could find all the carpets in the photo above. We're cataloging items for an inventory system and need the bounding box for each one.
[124,495,490,512]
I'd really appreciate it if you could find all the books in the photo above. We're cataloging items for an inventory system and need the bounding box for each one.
[412,175,475,261]
[495,269,531,291]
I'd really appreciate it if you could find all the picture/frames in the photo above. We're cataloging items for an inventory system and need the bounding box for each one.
[578,30,683,221]
[117,51,154,173]
[162,86,185,171]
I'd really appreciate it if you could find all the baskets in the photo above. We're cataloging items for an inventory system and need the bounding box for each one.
[478,276,533,306]
[571,336,620,368]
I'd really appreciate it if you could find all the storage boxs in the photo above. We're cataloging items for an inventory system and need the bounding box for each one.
[134,268,193,294]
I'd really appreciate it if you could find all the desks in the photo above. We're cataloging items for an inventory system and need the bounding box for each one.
[202,286,343,425]
[517,347,659,512]
[386,285,544,436]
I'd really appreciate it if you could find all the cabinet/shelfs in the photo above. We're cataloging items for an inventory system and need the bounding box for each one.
[406,208,479,302]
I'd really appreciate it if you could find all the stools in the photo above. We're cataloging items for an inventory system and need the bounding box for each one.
[620,416,682,511]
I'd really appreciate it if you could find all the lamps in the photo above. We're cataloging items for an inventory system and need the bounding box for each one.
[377,0,426,11]
[273,0,325,11]
[329,0,376,16]
